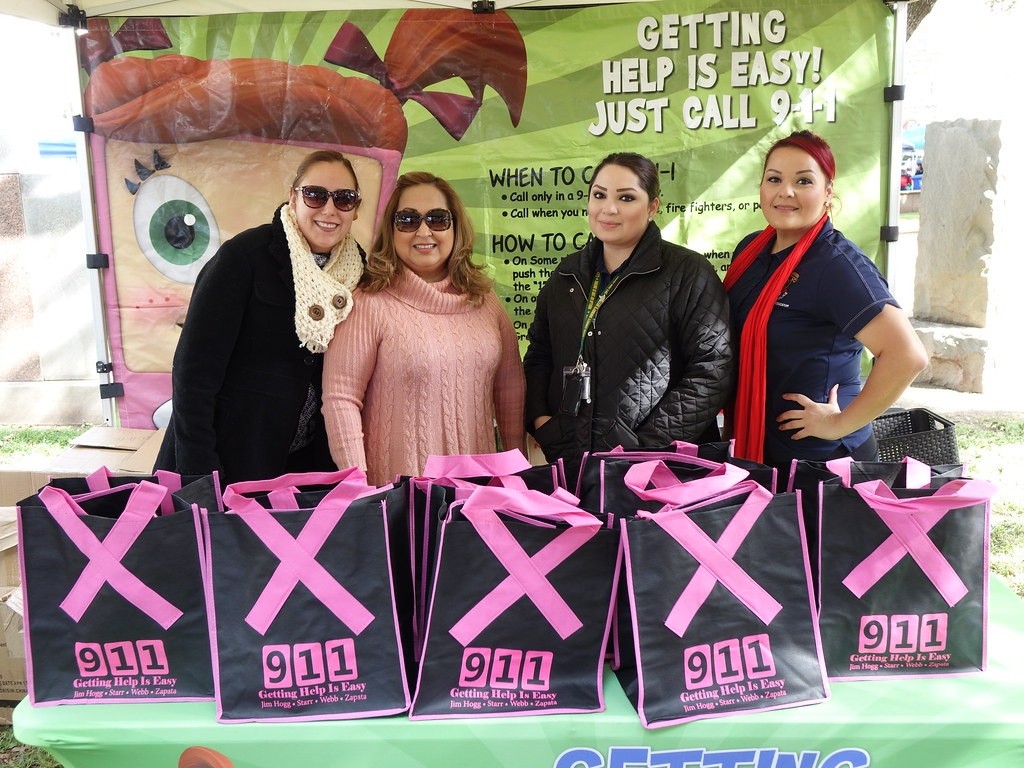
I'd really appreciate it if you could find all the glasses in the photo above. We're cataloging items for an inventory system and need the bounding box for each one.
[294,186,361,211]
[393,209,454,233]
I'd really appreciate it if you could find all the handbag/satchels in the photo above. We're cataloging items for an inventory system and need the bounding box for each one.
[16,441,994,729]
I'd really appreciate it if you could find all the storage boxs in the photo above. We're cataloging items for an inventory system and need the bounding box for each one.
[0,425,171,701]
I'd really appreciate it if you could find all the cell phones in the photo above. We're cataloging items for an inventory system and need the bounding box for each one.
[559,374,583,417]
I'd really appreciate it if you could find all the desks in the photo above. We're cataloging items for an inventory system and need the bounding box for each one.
[11,570,1024,768]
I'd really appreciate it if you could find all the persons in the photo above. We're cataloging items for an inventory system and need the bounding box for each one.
[321,171,527,485]
[523,152,734,484]
[153,150,368,493]
[915,163,923,174]
[722,131,928,478]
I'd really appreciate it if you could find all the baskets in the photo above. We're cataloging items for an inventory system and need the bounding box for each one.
[870,407,959,466]
